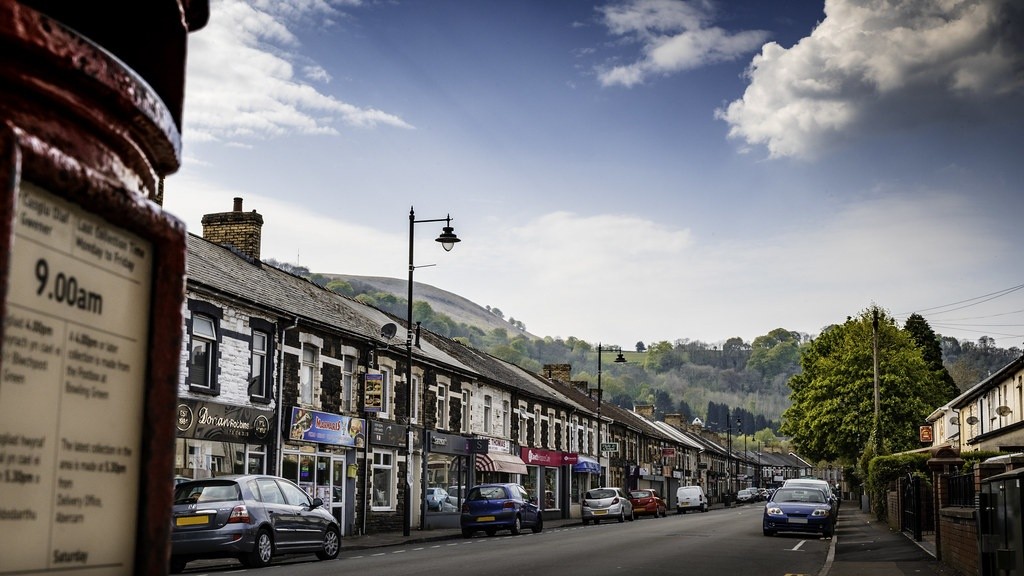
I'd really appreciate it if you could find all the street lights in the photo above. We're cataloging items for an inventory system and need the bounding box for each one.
[726,412,743,493]
[597,343,627,487]
[401,206,464,539]
[744,433,755,476]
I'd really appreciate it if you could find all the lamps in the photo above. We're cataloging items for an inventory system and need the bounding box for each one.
[966,416,979,425]
[949,417,961,426]
[996,406,1012,416]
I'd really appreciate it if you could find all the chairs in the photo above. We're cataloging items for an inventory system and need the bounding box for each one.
[781,492,792,500]
[809,494,818,501]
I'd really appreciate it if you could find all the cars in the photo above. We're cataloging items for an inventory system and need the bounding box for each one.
[676,485,710,513]
[761,485,837,538]
[747,487,776,501]
[582,488,635,525]
[170,474,343,570]
[784,479,837,511]
[629,488,669,518]
[428,484,466,514]
[735,490,753,504]
[459,482,546,535]
